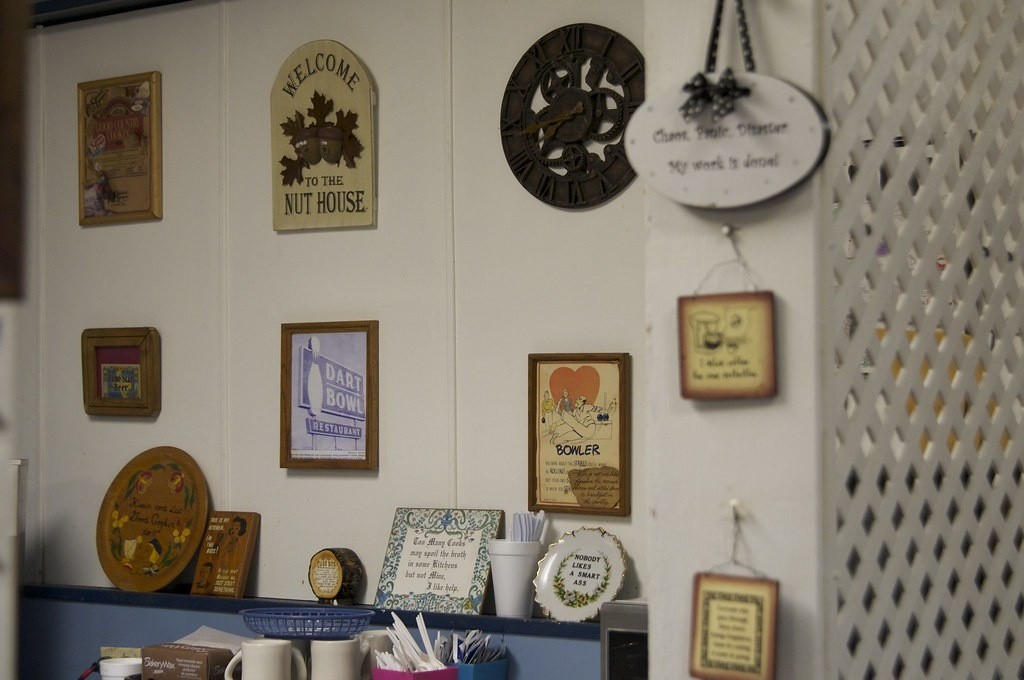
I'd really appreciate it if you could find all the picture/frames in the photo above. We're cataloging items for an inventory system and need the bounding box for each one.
[527,352,633,517]
[76,70,163,226]
[280,321,379,472]
[80,326,163,418]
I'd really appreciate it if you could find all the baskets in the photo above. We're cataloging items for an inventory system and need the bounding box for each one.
[238,605,376,640]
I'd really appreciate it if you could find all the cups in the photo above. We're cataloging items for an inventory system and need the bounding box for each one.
[224,628,399,680]
[99,657,142,680]
[489,538,541,618]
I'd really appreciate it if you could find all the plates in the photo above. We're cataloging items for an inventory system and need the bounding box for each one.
[96,446,209,592]
[533,526,627,622]
